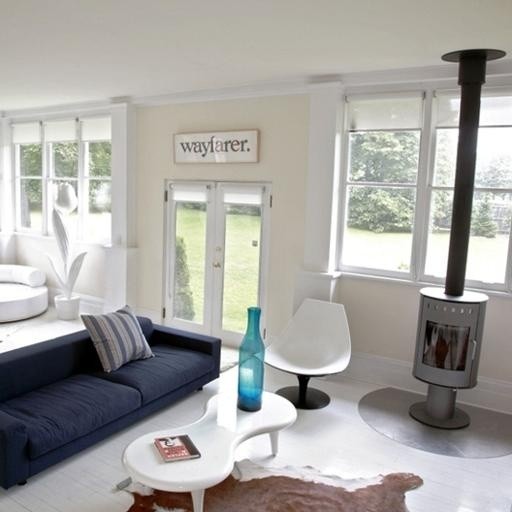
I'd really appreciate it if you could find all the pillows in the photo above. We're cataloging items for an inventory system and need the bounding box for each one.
[82,305,155,371]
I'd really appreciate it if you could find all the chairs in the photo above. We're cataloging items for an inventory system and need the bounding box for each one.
[256,296,352,411]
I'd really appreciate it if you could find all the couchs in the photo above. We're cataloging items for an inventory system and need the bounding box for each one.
[0,265,49,323]
[0,313,221,488]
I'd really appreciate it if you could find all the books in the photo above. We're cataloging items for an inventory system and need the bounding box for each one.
[155,435,201,463]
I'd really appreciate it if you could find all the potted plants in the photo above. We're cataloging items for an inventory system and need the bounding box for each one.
[47,207,87,319]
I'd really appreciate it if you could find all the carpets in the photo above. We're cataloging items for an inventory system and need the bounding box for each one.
[116,455,422,512]
